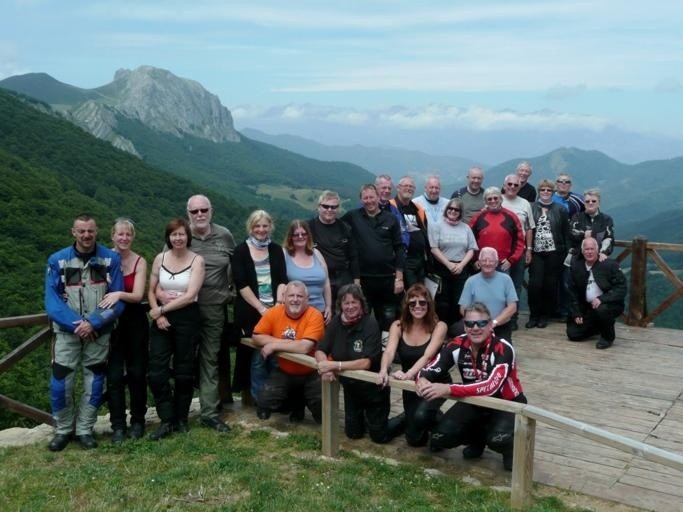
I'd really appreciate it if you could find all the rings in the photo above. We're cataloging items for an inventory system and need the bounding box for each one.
[399,375,402,378]
[107,302,110,304]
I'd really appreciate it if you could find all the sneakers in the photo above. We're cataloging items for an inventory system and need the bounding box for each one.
[463,446,512,470]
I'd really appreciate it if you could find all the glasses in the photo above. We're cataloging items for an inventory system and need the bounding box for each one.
[487,197,498,201]
[408,300,427,307]
[448,207,460,211]
[464,319,489,327]
[541,188,551,192]
[321,203,339,209]
[557,179,570,183]
[585,199,596,203]
[189,208,209,214]
[508,183,518,187]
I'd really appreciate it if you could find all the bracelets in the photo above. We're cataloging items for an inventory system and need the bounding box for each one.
[275,300,285,304]
[160,304,164,315]
[338,361,342,372]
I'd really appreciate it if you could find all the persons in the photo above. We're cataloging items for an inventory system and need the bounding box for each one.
[375,281,448,452]
[45,215,126,453]
[414,300,528,471]
[280,159,628,350]
[314,283,405,445]
[251,280,326,426]
[154,193,238,435]
[145,217,205,441]
[230,207,291,407]
[61,214,150,441]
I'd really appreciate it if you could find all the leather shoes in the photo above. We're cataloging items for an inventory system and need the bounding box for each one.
[509,314,569,332]
[49,434,72,451]
[202,417,229,432]
[79,433,97,448]
[257,407,270,419]
[597,337,611,348]
[111,419,190,445]
